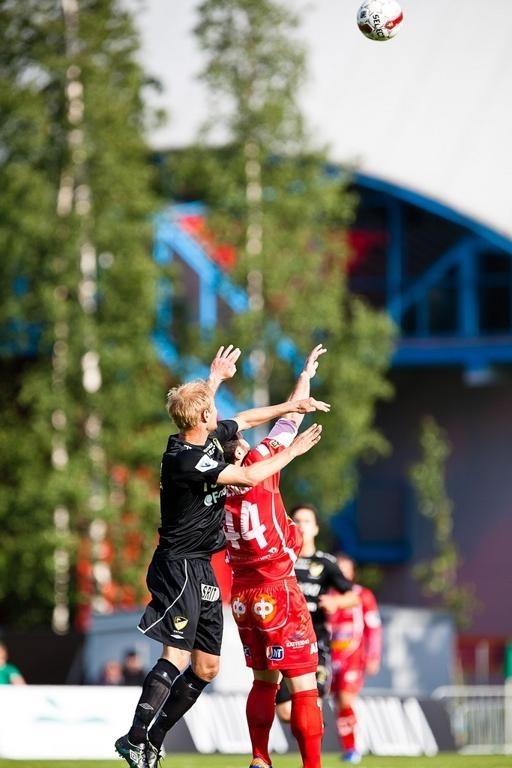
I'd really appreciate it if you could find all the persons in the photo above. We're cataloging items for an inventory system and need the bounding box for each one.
[101,658,124,685]
[122,648,145,682]
[276,502,362,759]
[202,343,330,768]
[319,552,381,760]
[115,378,332,768]
[0,646,24,686]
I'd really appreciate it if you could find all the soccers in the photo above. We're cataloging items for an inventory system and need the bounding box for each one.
[356,0,404,42]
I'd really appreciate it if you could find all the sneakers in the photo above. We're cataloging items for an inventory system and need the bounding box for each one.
[341,749,363,764]
[249,758,273,768]
[115,733,165,768]
